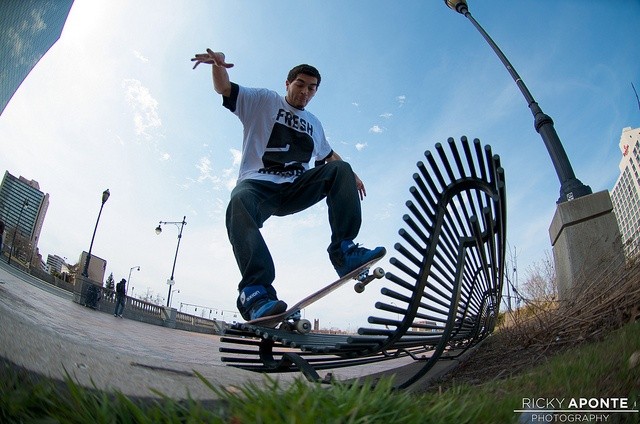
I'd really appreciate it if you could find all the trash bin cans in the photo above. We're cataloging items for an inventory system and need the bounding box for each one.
[85,284,98,309]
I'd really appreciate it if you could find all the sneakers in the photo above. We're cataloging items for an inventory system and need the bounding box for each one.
[328,240,386,280]
[239,285,287,320]
[118,314,123,318]
[113,314,117,317]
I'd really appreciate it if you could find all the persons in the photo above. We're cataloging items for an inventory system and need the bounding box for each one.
[190,48,387,321]
[113,278,127,319]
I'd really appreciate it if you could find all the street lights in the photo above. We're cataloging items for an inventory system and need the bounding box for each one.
[80,188,110,279]
[155,216,188,307]
[445,0,593,206]
[7,198,29,265]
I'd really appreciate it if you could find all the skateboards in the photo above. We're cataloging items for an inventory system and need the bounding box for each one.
[245,255,385,333]
